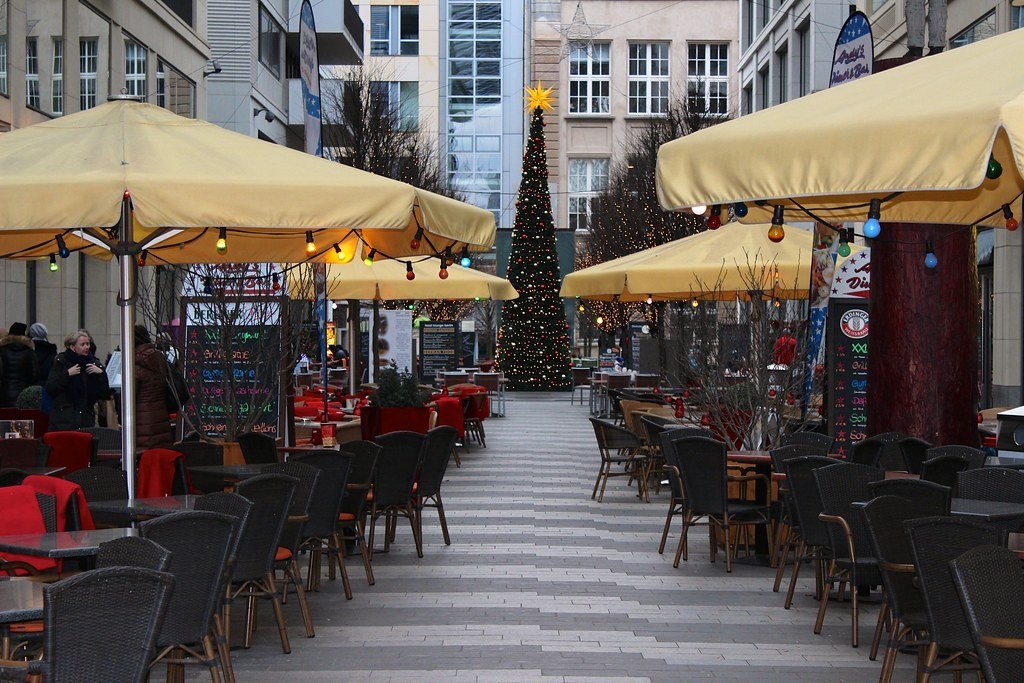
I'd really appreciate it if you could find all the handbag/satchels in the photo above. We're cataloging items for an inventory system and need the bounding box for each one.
[164,361,190,414]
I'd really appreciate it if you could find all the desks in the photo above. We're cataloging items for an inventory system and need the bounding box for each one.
[0,579,55,623]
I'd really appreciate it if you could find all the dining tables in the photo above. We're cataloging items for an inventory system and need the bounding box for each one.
[187,463,272,490]
[726,451,796,567]
[84,493,200,516]
[27,465,66,475]
[952,497,1024,551]
[0,528,140,570]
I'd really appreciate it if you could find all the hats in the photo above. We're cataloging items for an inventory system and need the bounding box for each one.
[29,324,47,339]
[9,322,27,336]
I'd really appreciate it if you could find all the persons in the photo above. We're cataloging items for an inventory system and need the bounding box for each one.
[336,345,350,365]
[0,321,179,430]
[773,329,798,404]
[133,324,189,454]
[329,345,346,367]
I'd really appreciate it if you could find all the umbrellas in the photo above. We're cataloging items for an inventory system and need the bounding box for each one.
[0,89,495,499]
[559,221,866,302]
[655,23,1024,228]
[281,237,518,379]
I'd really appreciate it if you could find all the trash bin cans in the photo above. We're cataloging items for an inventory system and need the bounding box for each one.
[996,406,1024,558]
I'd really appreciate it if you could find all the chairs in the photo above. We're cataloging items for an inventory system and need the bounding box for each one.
[573,368,1024,682]
[0,368,506,682]
